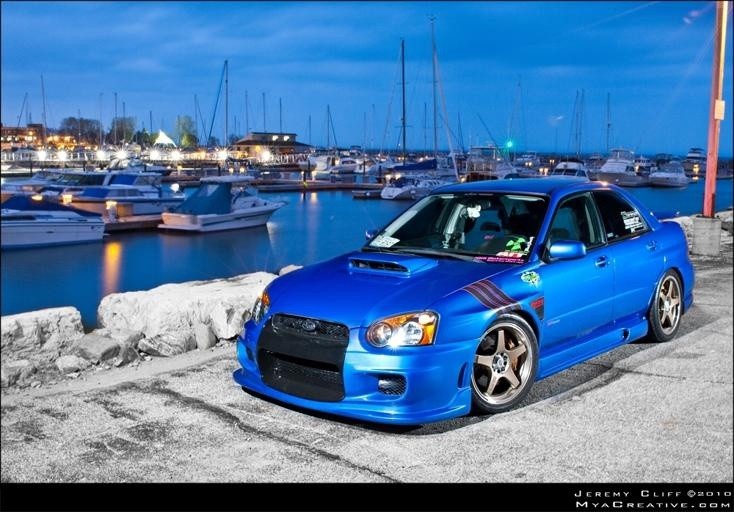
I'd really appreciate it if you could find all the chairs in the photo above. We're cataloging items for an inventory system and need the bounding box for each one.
[462,195,626,250]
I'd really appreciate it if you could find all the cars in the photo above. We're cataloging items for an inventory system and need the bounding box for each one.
[233,175,695,425]
